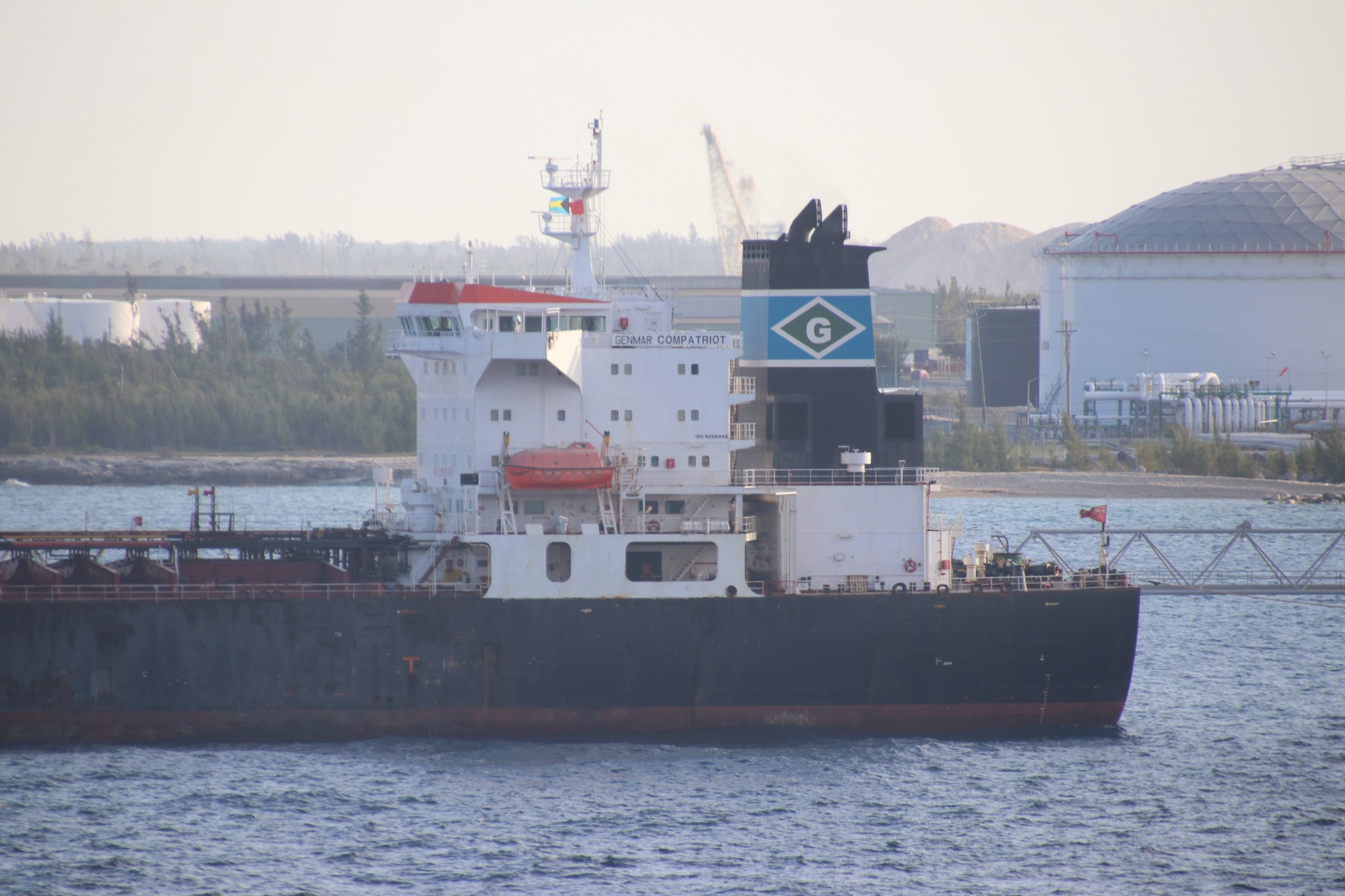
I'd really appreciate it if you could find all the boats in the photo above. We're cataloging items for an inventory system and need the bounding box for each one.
[499,439,616,493]
[0,116,1143,749]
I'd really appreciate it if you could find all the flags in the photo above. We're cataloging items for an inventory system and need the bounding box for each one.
[567,199,584,214]
[549,195,569,214]
[1080,505,1107,524]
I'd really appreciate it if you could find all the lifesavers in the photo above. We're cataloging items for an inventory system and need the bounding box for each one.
[905,560,917,573]
[648,521,660,532]
[481,576,491,591]
[937,584,949,594]
[893,583,907,596]
[971,583,983,594]
[1001,582,1013,593]
